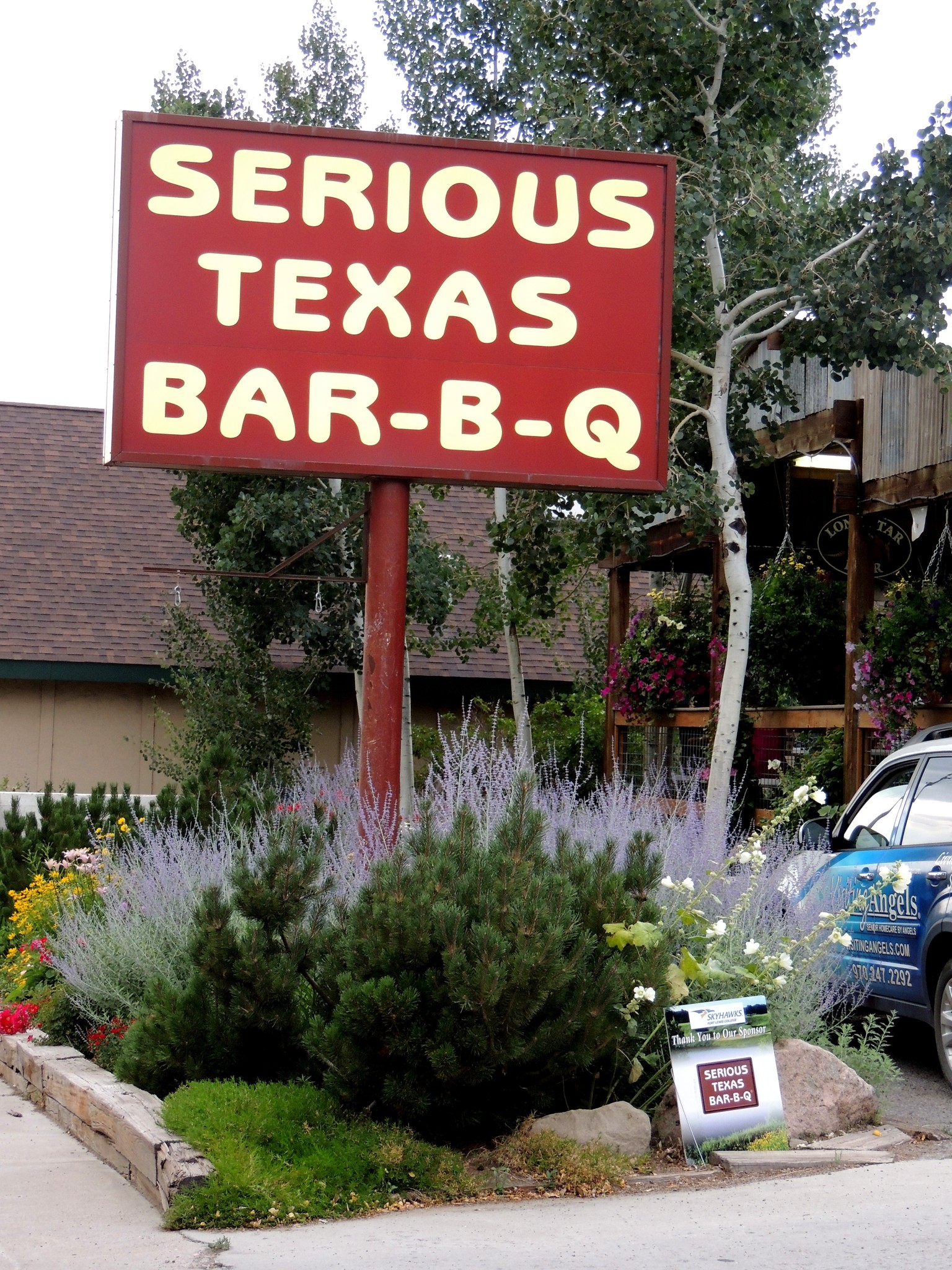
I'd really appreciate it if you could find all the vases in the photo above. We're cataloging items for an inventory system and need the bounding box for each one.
[752,728,788,776]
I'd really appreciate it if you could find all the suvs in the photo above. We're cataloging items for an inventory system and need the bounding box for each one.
[759,722,952,1090]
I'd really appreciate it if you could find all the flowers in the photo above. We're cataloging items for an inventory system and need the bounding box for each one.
[601,543,952,758]
